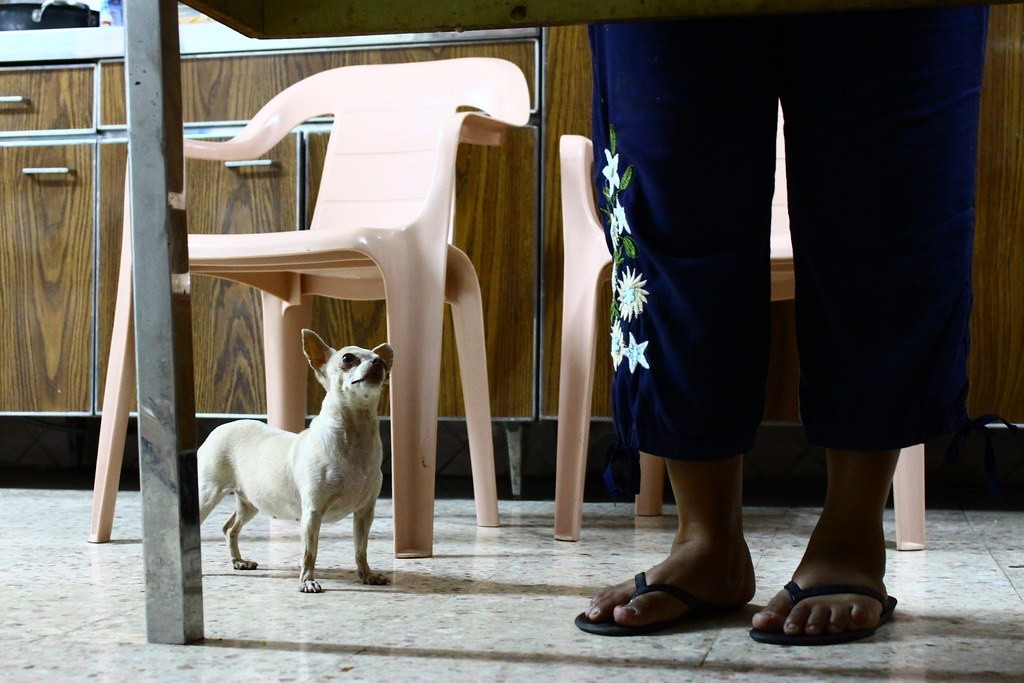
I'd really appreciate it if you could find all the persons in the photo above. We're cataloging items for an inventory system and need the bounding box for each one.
[574,0,991,646]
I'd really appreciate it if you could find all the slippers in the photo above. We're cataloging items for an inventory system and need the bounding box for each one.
[749,581,897,646]
[575,572,755,635]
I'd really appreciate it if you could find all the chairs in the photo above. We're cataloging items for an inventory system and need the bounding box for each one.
[83,56,534,559]
[551,89,927,553]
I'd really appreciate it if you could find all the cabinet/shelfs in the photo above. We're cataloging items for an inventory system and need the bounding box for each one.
[0,1,1024,498]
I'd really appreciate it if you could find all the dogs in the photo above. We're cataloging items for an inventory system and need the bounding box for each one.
[197,327,394,596]
